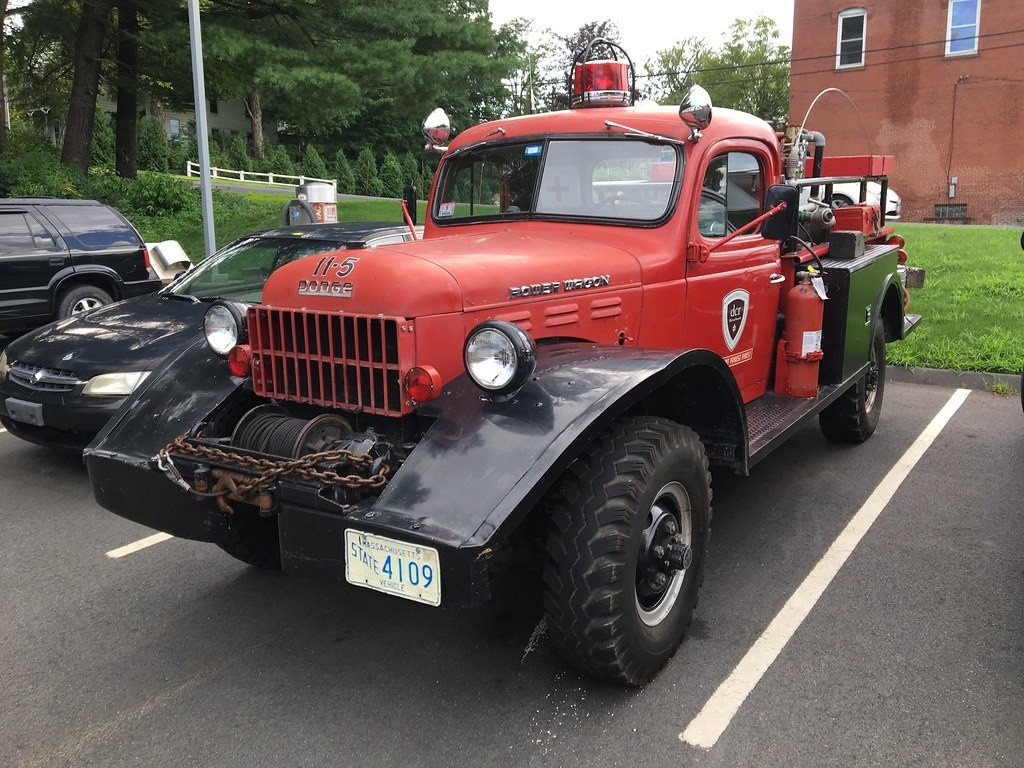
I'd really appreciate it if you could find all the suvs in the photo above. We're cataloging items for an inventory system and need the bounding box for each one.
[0,197,161,344]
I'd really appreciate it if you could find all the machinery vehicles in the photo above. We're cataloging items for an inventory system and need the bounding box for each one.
[83,32,925,691]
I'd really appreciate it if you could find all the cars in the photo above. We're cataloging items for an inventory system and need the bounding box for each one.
[799,174,904,222]
[0,222,425,456]
